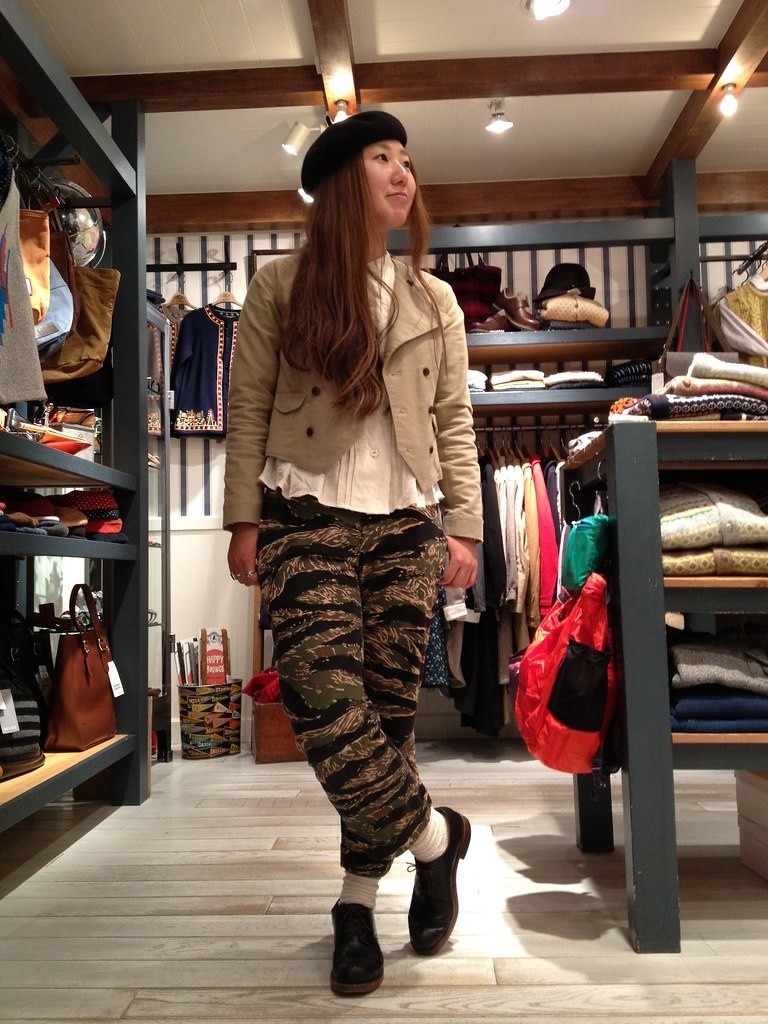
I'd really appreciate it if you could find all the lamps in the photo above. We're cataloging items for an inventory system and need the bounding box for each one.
[281,121,327,154]
[484,97,513,135]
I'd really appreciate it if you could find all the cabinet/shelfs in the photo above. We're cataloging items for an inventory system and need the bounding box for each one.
[0,2,146,902]
[395,212,768,415]
[560,416,768,954]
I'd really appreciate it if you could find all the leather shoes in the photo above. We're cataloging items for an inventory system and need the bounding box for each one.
[469,308,523,333]
[407,806,471,955]
[329,895,384,994]
[493,288,540,331]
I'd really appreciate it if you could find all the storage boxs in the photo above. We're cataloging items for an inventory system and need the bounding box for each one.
[732,768,768,884]
[249,697,307,765]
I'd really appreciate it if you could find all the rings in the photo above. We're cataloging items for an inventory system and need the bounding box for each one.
[245,570,255,577]
[230,572,240,580]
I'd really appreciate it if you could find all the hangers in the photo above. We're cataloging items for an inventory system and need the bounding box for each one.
[731,242,768,287]
[472,424,569,472]
[164,271,197,310]
[210,271,244,309]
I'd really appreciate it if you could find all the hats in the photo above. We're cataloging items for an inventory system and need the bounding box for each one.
[533,262,596,305]
[301,111,408,193]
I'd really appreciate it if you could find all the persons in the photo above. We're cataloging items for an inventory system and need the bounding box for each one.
[221,110,484,997]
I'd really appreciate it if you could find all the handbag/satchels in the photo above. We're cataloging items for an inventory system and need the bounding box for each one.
[651,280,743,400]
[421,243,503,333]
[513,495,616,775]
[0,584,121,782]
[18,184,121,381]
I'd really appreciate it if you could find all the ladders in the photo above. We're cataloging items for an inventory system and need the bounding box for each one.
[87,301,175,759]
